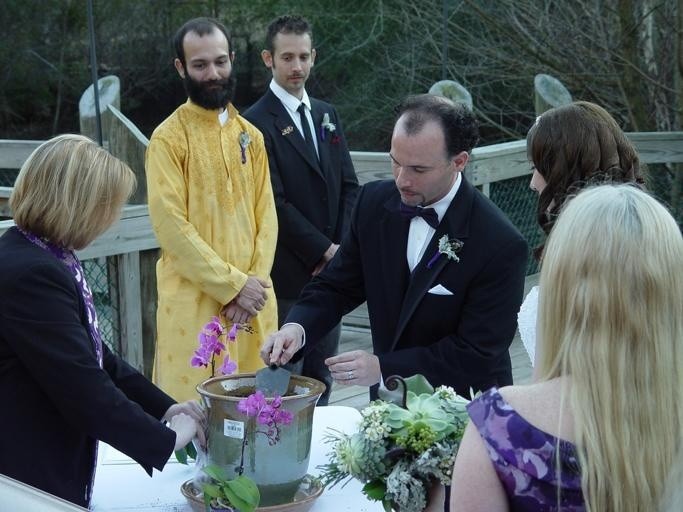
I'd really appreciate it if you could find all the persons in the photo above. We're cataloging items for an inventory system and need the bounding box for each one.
[447,180,682,512]
[0,133,207,507]
[242,14,362,367]
[143,17,284,408]
[513,100,642,368]
[258,93,528,406]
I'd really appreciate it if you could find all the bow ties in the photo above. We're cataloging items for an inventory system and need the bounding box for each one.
[400,203,439,231]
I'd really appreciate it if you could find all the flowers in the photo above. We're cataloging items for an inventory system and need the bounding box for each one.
[423,230,468,269]
[310,372,484,512]
[173,315,255,465]
[199,390,297,511]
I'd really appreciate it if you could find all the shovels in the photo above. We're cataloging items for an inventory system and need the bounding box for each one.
[254,348,291,398]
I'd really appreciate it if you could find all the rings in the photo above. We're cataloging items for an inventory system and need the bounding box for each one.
[348,370,353,379]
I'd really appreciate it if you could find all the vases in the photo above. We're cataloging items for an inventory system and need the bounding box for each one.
[192,372,327,509]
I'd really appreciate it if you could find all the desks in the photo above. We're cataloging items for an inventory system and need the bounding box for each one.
[89,402,399,512]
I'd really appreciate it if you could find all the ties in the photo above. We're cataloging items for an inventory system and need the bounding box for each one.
[297,103,320,168]
